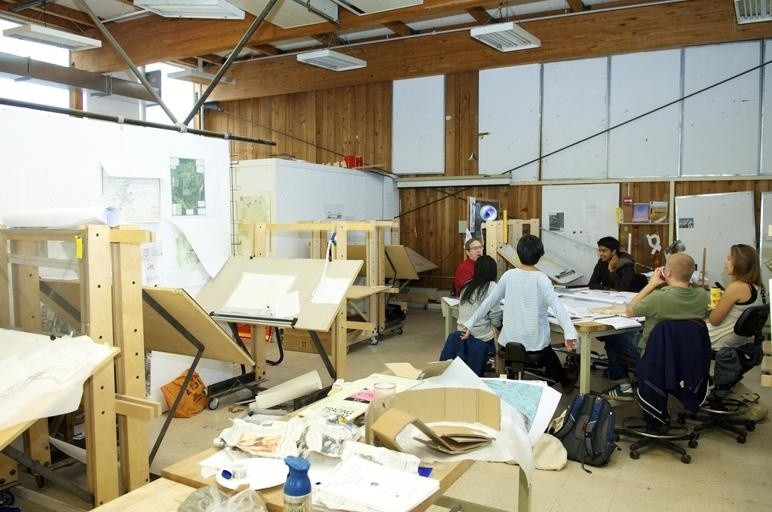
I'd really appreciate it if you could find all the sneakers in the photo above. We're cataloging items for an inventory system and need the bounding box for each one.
[564,374,578,394]
[602,383,634,402]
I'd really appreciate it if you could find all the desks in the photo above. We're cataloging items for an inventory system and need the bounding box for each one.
[160,374,536,512]
[446,289,647,397]
[84,476,277,512]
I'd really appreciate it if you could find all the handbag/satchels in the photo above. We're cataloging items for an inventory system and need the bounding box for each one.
[161,368,208,417]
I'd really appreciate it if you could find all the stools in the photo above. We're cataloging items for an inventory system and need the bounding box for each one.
[498,341,559,385]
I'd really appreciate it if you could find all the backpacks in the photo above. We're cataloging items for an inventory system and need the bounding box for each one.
[546,391,621,474]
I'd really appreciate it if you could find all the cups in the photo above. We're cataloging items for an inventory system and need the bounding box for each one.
[711,287,724,307]
[374,382,397,401]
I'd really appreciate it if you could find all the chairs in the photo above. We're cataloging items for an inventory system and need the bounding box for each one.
[677,302,771,448]
[612,321,709,465]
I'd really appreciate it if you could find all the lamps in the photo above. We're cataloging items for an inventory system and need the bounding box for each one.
[468,1,540,53]
[132,0,246,21]
[296,24,367,72]
[733,0,772,25]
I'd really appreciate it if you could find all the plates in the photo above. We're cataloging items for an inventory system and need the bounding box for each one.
[217,457,292,491]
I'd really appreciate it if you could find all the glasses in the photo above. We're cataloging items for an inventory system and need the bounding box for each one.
[466,246,484,250]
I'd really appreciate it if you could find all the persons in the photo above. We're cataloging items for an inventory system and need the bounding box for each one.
[455,238,483,297]
[588,236,635,292]
[706,244,766,352]
[460,235,579,395]
[600,253,709,402]
[456,255,504,360]
